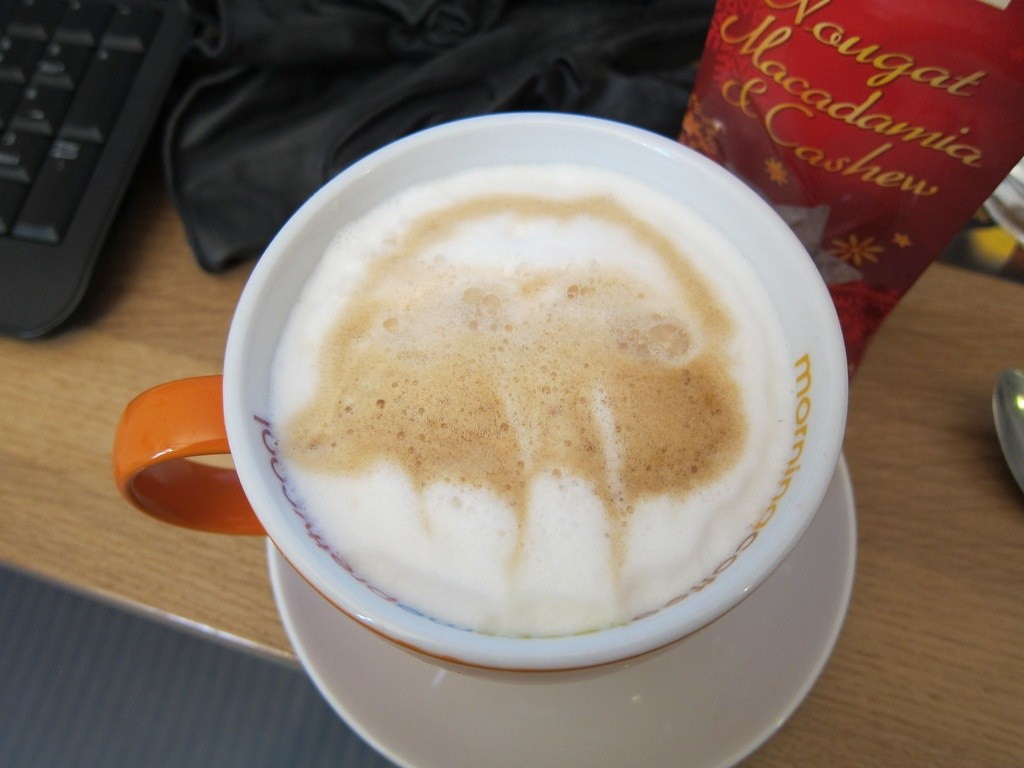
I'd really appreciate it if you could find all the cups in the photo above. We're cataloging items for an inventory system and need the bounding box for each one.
[114,111,848,668]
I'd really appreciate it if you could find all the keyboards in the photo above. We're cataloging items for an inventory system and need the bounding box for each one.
[0,0,192,342]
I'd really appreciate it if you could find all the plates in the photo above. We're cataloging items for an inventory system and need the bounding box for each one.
[268,454,855,768]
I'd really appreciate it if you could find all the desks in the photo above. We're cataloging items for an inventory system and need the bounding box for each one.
[0,213,1024,768]
